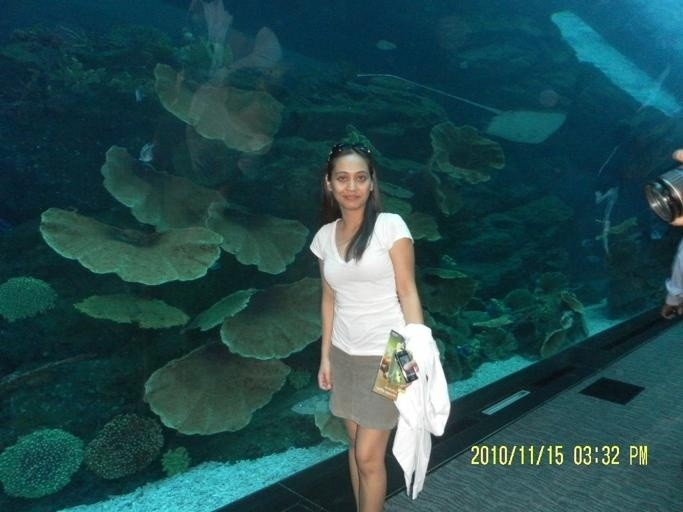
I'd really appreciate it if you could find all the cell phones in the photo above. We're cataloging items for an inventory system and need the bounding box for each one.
[395,351,418,383]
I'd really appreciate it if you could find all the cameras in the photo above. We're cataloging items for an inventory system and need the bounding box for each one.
[645,165,683,224]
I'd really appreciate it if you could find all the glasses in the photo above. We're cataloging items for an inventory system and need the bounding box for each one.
[327,143,371,161]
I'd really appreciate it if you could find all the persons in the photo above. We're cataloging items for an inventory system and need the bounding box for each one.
[308,142,425,512]
[661,237,683,321]
[661,146,683,225]
